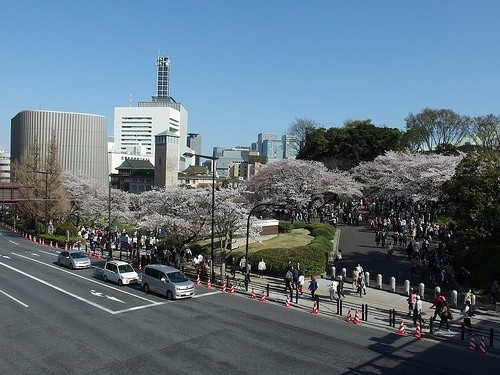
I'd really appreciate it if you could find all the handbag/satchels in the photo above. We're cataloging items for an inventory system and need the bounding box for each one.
[448,310,454,320]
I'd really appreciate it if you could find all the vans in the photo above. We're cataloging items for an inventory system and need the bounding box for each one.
[144,265,195,300]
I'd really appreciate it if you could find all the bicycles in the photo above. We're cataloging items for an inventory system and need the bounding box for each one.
[416,252,449,290]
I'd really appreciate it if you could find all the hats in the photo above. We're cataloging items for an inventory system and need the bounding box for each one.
[310,275,316,280]
[416,295,420,300]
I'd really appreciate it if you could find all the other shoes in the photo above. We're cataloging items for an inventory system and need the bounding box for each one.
[339,297,342,299]
[333,297,337,300]
[357,291,359,293]
[343,295,345,298]
[432,317,435,321]
[364,292,366,295]
[412,324,417,327]
[439,329,441,331]
[422,320,427,326]
[447,329,450,332]
[408,312,411,315]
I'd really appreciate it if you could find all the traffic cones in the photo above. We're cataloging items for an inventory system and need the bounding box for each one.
[311,300,319,315]
[220,281,228,292]
[477,335,488,354]
[283,295,294,307]
[259,291,268,302]
[1,223,102,259]
[351,309,361,325]
[228,282,236,294]
[247,287,257,300]
[205,277,212,289]
[193,274,202,286]
[128,260,133,267]
[395,319,408,336]
[137,262,142,271]
[414,321,422,339]
[345,307,353,322]
[465,333,478,352]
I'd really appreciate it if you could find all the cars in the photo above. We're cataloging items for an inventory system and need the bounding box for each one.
[102,260,139,287]
[58,250,91,270]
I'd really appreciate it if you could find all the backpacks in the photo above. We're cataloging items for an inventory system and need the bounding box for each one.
[466,304,475,318]
[413,301,419,319]
[434,299,447,311]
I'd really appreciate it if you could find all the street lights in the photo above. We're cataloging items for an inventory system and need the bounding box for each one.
[26,170,52,233]
[182,151,220,283]
[245,203,288,290]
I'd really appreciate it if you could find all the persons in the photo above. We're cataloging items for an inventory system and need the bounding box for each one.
[356,263,366,298]
[430,292,452,333]
[292,200,369,227]
[327,275,347,301]
[369,198,456,285]
[407,290,426,326]
[283,265,317,299]
[461,291,476,328]
[77,222,185,267]
[231,255,268,280]
[186,247,204,266]
